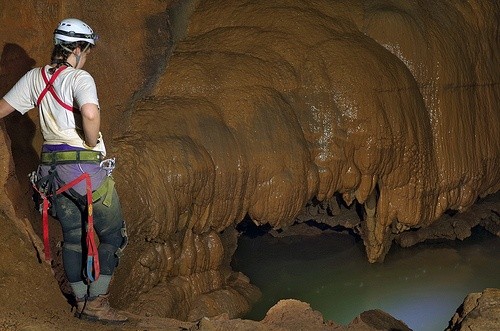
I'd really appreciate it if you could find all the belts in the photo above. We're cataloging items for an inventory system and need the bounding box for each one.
[41,151,101,163]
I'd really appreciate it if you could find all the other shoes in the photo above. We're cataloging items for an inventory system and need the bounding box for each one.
[83,292,129,323]
[76,294,88,319]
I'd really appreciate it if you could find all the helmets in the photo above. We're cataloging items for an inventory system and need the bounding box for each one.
[53,18,99,46]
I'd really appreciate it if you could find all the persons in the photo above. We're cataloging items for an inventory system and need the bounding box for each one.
[0,17,131,323]
